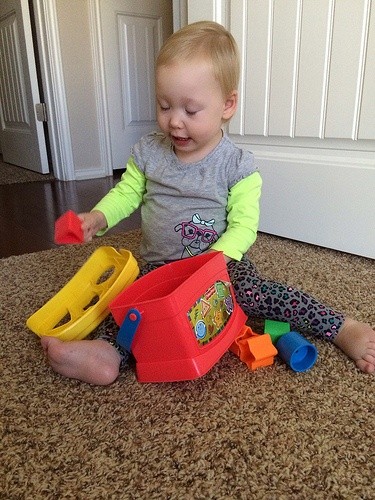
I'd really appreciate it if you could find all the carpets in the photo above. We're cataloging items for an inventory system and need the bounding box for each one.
[0,227,375,500]
[0,159,55,186]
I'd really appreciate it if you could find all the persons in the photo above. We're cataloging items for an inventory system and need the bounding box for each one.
[40,21,375,386]
